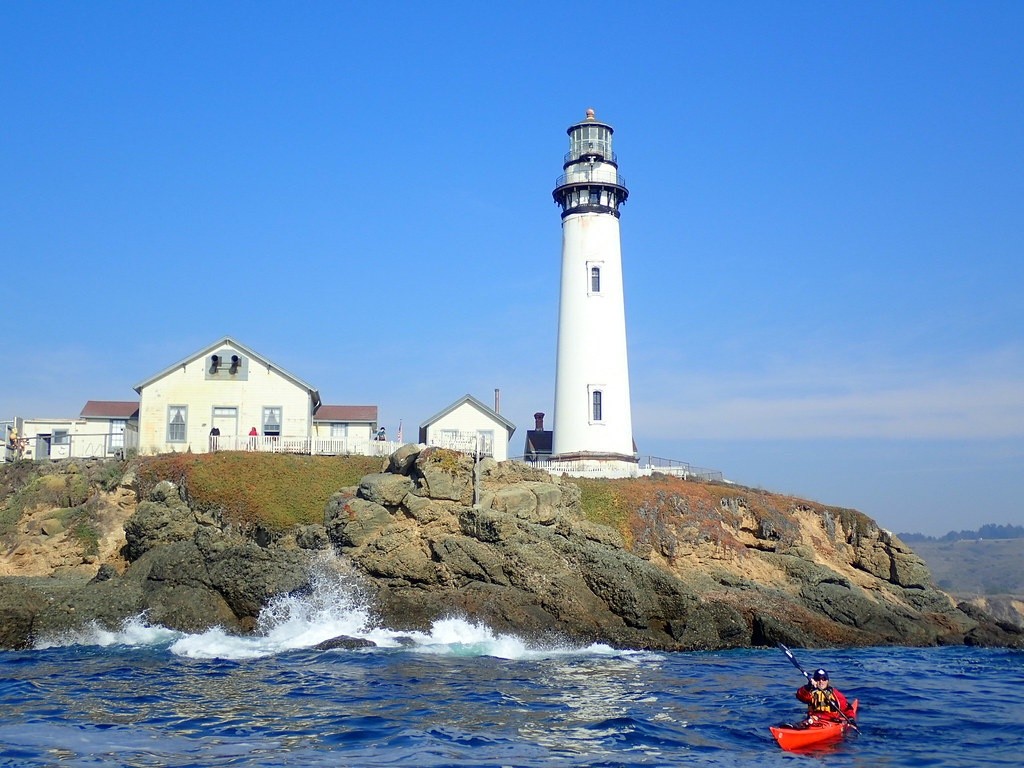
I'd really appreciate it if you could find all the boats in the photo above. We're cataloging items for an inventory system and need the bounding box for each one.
[769,697,859,751]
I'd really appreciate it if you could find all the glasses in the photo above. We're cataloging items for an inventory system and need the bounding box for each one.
[814,677,828,681]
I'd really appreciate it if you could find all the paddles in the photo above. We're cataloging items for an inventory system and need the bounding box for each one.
[776,641,862,735]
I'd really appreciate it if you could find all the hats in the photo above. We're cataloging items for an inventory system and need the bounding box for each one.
[813,668,829,680]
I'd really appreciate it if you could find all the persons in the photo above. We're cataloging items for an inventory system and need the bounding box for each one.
[210,426,220,449]
[10,428,24,459]
[249,426,259,449]
[374,427,386,441]
[783,668,859,730]
